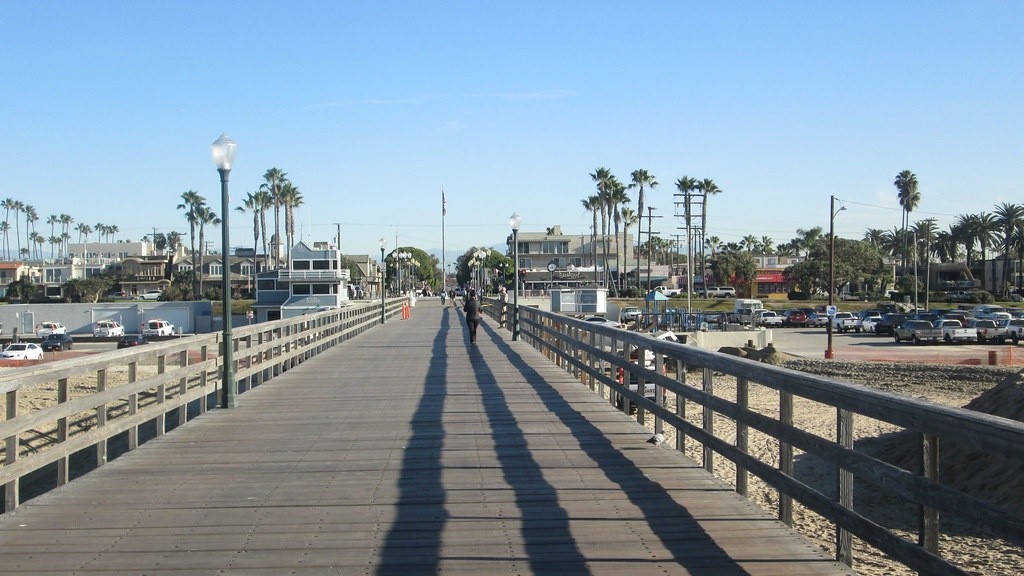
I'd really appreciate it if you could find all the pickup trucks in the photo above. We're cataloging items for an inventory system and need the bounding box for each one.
[831,311,863,334]
[931,318,978,345]
[647,285,682,299]
[969,318,1008,346]
[894,318,945,346]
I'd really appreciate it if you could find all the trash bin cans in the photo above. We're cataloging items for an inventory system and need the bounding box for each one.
[408,296,416,307]
[13,335,20,343]
[988,351,999,365]
[748,340,753,347]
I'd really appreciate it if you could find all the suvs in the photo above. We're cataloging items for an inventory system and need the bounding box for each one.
[35,321,66,339]
[698,286,737,299]
[94,320,125,337]
[141,320,175,341]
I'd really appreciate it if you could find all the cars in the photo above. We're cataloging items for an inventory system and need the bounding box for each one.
[41,333,73,351]
[116,334,148,350]
[138,289,164,301]
[107,291,135,302]
[346,283,365,301]
[0,343,44,361]
[453,287,468,296]
[999,317,1024,345]
[854,300,1024,337]
[554,304,830,413]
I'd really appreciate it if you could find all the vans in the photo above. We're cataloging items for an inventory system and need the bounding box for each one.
[734,298,764,310]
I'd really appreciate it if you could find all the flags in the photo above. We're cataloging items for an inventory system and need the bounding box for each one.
[443,192,446,216]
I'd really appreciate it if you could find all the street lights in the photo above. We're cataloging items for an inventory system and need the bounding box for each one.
[378,237,389,324]
[508,211,522,341]
[391,251,398,298]
[205,129,240,411]
[830,205,848,307]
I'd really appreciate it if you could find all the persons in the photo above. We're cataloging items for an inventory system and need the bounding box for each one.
[464,290,481,347]
[449,287,456,305]
[423,288,432,297]
[440,289,446,305]
[497,287,508,329]
